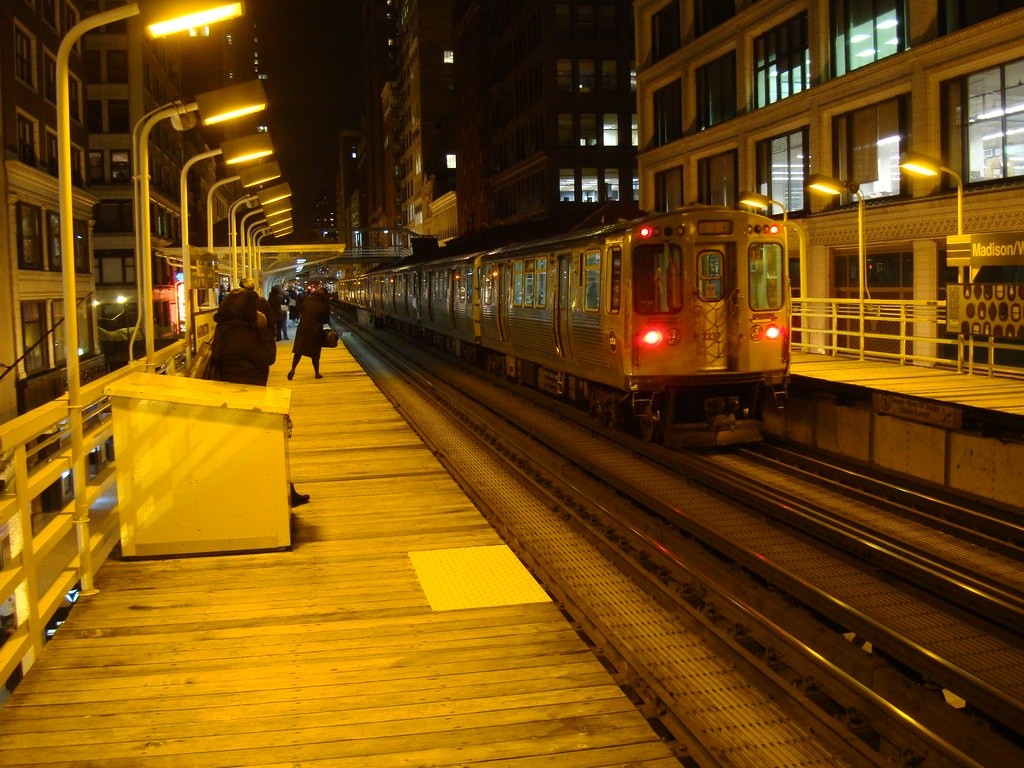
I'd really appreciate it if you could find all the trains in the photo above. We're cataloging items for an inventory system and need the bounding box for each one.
[326,204,790,450]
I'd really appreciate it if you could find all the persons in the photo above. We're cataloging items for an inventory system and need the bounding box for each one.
[268,281,307,342]
[240,279,277,338]
[287,288,330,380]
[214,289,309,508]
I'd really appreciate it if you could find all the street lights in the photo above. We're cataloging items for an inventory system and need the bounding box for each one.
[744,195,788,228]
[139,80,267,374]
[53,0,243,597]
[182,134,295,359]
[805,172,868,361]
[895,158,964,372]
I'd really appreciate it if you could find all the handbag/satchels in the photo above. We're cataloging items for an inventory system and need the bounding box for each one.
[185,335,213,379]
[321,320,339,348]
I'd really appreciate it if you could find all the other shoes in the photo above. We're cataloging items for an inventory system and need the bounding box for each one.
[276,337,283,341]
[284,337,289,340]
[290,482,310,508]
[315,373,322,379]
[288,369,295,380]
